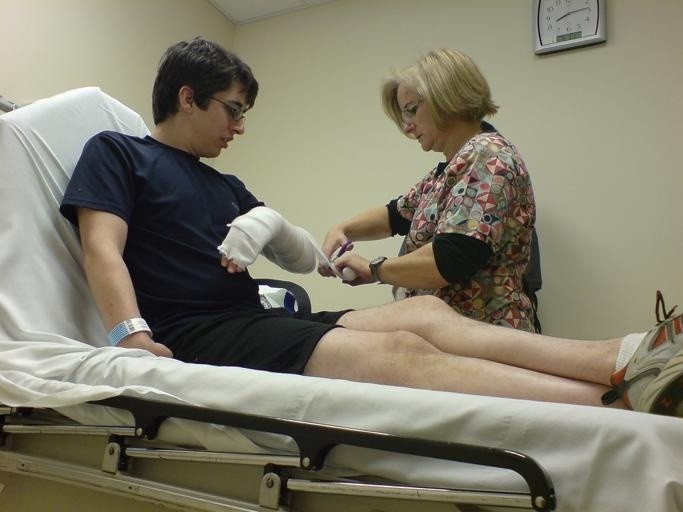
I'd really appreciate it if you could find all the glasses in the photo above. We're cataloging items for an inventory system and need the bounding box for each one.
[400,102,420,129]
[208,96,247,121]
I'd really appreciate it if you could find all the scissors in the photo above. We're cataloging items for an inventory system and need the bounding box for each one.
[331,239,353,262]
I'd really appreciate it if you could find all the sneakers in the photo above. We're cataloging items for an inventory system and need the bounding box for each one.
[601,291,683,412]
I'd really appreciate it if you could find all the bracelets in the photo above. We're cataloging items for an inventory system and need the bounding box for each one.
[107,318,153,347]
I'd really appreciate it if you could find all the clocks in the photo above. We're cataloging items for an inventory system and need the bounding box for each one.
[530,0,608,55]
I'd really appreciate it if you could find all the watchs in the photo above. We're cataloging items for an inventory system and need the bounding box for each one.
[369,256,387,285]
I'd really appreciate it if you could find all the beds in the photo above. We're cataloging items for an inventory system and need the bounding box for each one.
[0,81,682,512]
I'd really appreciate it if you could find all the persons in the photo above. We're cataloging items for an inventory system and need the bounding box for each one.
[58,36,683,420]
[318,47,541,336]
